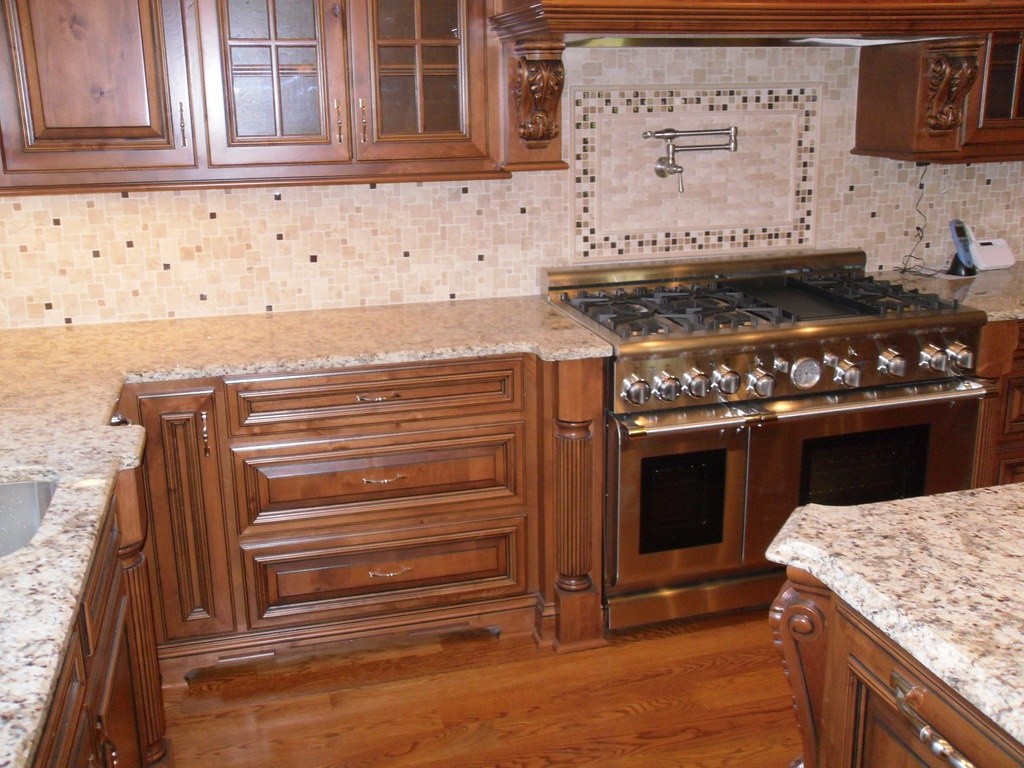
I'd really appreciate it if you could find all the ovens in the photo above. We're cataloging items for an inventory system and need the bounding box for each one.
[605,373,1000,632]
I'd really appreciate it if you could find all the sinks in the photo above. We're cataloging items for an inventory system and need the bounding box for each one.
[0,466,62,561]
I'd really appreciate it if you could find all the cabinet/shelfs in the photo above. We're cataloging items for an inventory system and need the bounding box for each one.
[957,29,1024,162]
[971,319,1024,489]
[221,352,543,661]
[0,0,203,196]
[30,493,145,768]
[192,0,514,191]
[119,376,249,673]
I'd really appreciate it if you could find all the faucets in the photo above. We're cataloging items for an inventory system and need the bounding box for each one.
[655,145,684,193]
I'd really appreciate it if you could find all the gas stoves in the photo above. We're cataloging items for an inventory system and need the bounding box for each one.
[538,247,990,417]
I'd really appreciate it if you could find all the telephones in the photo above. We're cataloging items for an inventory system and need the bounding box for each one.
[946,218,977,277]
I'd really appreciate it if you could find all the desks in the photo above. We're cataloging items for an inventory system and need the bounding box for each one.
[762,482,1023,768]
